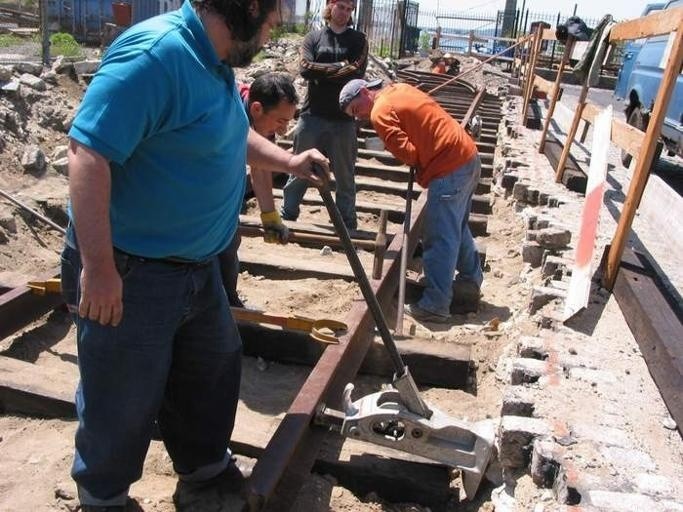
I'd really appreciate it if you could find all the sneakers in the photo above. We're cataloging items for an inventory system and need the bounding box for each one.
[395,302,448,324]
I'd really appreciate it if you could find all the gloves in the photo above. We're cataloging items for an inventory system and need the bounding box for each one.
[260,210,289,245]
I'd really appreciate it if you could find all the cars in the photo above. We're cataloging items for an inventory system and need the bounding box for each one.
[614,2,682,169]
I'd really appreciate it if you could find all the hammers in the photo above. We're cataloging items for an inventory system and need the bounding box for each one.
[238,208,388,279]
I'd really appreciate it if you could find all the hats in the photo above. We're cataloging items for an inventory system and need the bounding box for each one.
[338,79,384,110]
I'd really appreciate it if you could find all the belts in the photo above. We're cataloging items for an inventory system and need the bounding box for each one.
[115,255,201,270]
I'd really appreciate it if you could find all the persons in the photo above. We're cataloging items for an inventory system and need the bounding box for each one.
[61,0,332,512]
[281,0,370,229]
[216,73,298,309]
[339,79,483,323]
